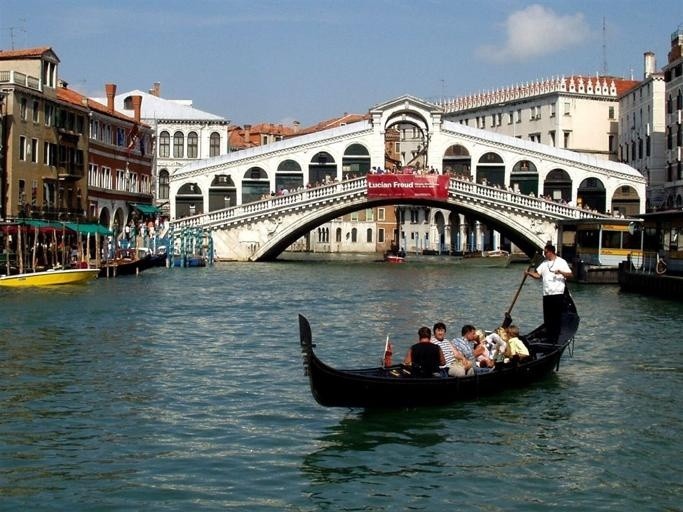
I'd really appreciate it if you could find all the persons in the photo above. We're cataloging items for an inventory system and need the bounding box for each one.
[524,243,576,345]
[403,321,533,378]
[30,236,91,270]
[124,212,166,243]
[390,240,405,257]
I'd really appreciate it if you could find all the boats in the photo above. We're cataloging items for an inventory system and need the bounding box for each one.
[298,280,580,411]
[69,246,153,279]
[384,255,406,264]
[292,373,561,508]
[612,207,682,299]
[156,253,206,268]
[459,249,511,269]
[0,268,101,288]
[553,218,647,285]
